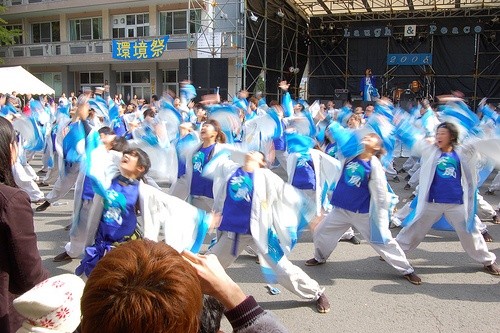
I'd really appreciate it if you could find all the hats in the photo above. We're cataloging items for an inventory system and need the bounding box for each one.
[13,273,85,333]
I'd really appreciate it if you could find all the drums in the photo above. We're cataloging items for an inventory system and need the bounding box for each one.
[395,88,404,100]
[410,81,420,92]
[400,90,416,102]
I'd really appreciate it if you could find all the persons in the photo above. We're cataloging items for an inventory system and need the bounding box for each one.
[0,67,500,312]
[0,115,53,333]
[79,237,283,333]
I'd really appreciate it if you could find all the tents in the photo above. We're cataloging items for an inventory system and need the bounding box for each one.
[0,66,55,99]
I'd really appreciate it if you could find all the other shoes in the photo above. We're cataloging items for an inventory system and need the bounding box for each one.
[493,215,500,224]
[404,272,422,285]
[392,155,415,200]
[379,256,386,262]
[53,251,70,262]
[483,232,495,242]
[31,198,45,203]
[485,190,494,196]
[65,223,72,230]
[37,181,50,187]
[36,171,48,176]
[350,235,361,245]
[316,293,331,313]
[305,258,327,266]
[36,201,50,211]
[483,262,500,276]
[255,256,259,264]
[389,221,396,229]
